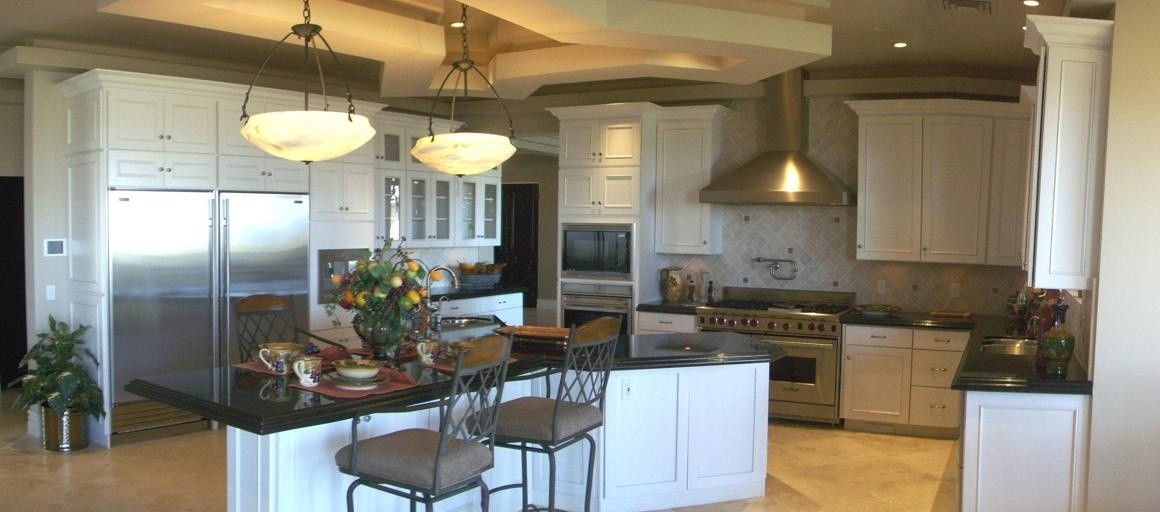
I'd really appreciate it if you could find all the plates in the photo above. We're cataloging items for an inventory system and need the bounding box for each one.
[853,303,903,318]
[325,371,390,391]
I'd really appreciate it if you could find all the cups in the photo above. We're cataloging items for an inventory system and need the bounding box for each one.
[258,348,293,375]
[416,338,440,365]
[259,375,290,403]
[415,367,438,388]
[291,355,324,388]
[1015,304,1025,321]
[290,391,322,411]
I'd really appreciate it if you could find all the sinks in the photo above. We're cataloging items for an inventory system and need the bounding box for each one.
[983,336,1026,340]
[978,341,1038,355]
[440,317,492,327]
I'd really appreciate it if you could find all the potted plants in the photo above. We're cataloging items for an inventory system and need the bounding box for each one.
[6,314,107,453]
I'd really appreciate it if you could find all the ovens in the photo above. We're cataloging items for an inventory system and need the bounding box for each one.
[697,329,840,426]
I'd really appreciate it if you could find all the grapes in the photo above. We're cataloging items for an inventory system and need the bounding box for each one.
[309,369,321,383]
[309,392,320,407]
[272,359,284,373]
[430,351,438,363]
[305,343,319,355]
[273,377,284,391]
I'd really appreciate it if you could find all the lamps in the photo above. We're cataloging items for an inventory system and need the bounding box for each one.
[239,0,377,163]
[409,5,519,177]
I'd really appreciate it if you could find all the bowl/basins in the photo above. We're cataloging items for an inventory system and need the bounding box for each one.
[256,342,304,368]
[460,261,504,276]
[332,358,383,379]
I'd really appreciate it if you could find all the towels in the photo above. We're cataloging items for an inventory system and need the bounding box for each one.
[233,341,519,401]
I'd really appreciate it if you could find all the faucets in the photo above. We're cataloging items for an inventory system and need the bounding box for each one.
[426,265,459,323]
[1026,316,1040,339]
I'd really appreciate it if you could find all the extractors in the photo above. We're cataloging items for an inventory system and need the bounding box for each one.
[697,68,858,207]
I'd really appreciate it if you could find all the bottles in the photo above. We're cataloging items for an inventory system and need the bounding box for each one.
[1006,297,1017,320]
[1034,300,1052,378]
[685,269,717,305]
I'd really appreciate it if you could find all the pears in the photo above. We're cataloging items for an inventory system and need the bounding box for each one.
[457,259,506,273]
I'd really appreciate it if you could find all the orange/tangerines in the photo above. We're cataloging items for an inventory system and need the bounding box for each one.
[331,258,443,311]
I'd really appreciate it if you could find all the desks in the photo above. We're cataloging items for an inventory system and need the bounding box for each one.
[124,307,777,512]
[427,282,529,343]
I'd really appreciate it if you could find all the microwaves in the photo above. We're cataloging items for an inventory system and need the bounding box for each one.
[562,224,632,280]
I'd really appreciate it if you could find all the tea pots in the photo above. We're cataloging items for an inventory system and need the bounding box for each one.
[659,265,684,303]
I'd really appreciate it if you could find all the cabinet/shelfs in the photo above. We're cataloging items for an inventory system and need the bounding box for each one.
[1019,43,1110,291]
[558,118,642,166]
[374,118,503,250]
[310,108,373,223]
[637,312,697,335]
[64,88,216,191]
[558,168,640,225]
[66,191,313,450]
[654,102,730,257]
[840,324,913,435]
[985,117,1029,267]
[857,99,995,266]
[216,94,309,194]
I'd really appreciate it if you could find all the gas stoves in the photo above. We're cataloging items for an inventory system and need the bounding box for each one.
[694,298,852,338]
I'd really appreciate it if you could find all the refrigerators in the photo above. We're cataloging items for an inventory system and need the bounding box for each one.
[106,187,312,432]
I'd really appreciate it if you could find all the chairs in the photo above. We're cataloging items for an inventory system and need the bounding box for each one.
[231,290,300,364]
[333,329,513,511]
[465,313,623,511]
